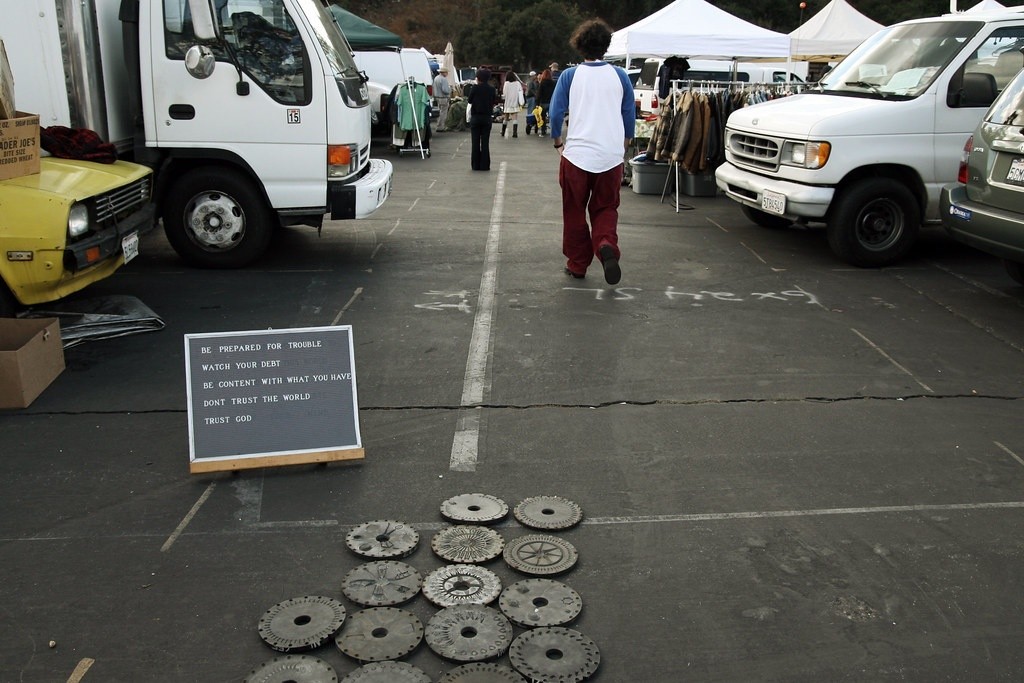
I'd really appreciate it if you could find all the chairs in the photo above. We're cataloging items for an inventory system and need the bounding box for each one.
[927,44,978,67]
[992,49,1024,90]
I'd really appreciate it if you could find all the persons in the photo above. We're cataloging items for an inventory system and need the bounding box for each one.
[525,62,562,137]
[432,65,449,131]
[500,71,524,137]
[548,18,635,284]
[466,69,495,170]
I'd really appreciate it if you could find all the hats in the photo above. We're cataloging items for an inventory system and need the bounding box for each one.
[437,66,450,72]
[529,71,536,75]
[548,62,558,67]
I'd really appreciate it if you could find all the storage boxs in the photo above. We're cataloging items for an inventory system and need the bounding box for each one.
[0,110,41,182]
[0,317,66,409]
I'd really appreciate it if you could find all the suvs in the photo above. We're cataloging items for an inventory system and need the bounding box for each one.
[715,12,1024,269]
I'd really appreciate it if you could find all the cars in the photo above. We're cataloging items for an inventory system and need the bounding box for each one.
[0,155,158,319]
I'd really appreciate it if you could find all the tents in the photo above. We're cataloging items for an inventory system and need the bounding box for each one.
[324,4,460,159]
[786,0,886,86]
[602,0,791,108]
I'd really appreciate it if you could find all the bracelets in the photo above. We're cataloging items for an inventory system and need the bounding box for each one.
[554,143,563,148]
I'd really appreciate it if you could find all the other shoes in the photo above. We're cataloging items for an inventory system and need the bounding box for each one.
[564,266,585,278]
[599,244,621,285]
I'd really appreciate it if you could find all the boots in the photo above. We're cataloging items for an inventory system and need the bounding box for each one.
[500,124,506,136]
[512,124,517,137]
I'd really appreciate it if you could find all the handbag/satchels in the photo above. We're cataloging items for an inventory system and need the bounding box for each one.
[465,85,475,123]
[518,82,525,105]
[533,106,544,128]
[526,114,537,125]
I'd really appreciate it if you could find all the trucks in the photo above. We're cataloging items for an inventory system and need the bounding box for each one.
[633,58,808,121]
[350,48,477,136]
[0,0,393,270]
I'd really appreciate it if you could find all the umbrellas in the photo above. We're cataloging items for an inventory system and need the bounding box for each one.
[441,41,461,108]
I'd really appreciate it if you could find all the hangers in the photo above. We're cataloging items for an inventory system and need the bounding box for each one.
[401,77,425,89]
[676,78,793,96]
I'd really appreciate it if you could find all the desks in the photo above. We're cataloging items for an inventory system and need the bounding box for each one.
[632,119,654,158]
